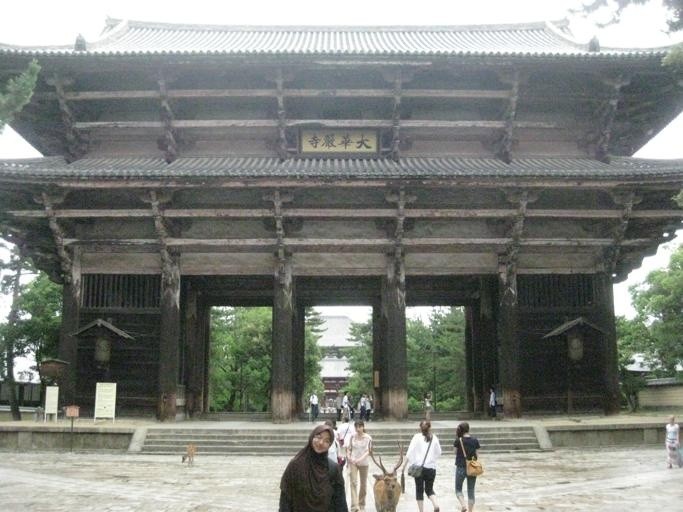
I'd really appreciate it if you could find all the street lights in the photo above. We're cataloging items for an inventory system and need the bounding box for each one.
[430,348,438,411]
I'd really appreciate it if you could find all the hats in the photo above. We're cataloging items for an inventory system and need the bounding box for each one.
[355,419,364,426]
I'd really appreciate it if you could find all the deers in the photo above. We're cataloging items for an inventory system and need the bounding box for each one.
[367,438,405,512]
[181,443,196,468]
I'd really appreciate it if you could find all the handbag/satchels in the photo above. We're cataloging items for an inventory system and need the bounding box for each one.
[466,460,483,476]
[337,439,344,446]
[408,465,423,477]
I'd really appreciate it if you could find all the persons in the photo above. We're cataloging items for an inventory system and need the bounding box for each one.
[489,387,496,421]
[424,391,433,420]
[309,393,319,419]
[665,415,683,469]
[360,393,372,422]
[454,422,481,512]
[335,391,354,423]
[402,420,440,512]
[278,416,372,512]
